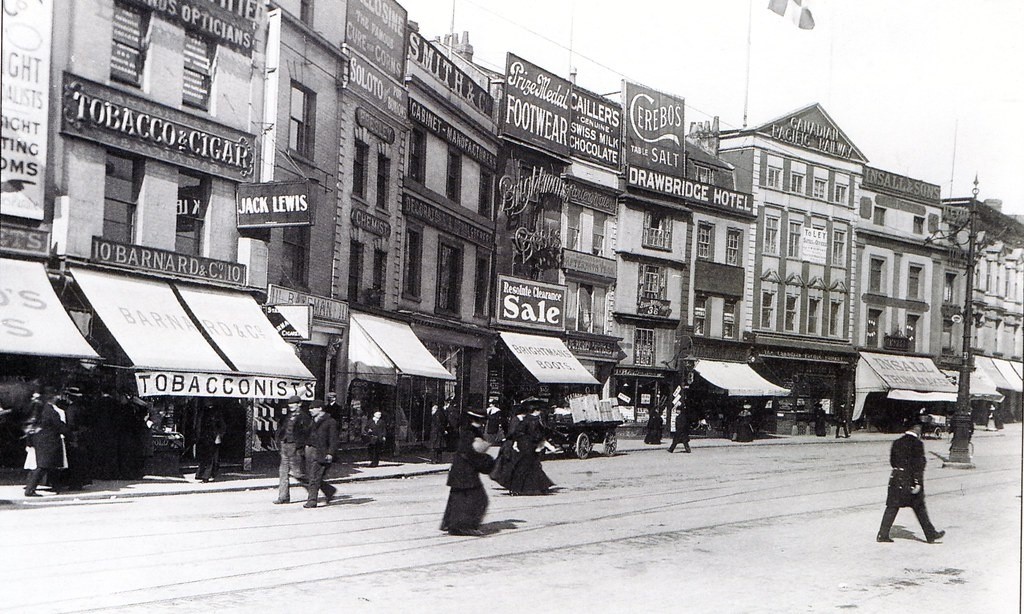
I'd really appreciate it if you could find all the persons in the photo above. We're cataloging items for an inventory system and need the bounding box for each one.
[877,417,945,545]
[0,370,1004,508]
[441,406,496,537]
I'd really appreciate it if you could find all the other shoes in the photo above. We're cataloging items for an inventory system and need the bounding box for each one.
[273,497,290,504]
[303,499,317,507]
[25,487,43,496]
[325,487,336,503]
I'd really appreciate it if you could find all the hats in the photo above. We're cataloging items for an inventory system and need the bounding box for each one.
[287,396,301,404]
[65,387,82,396]
[309,398,324,409]
[468,411,485,422]
[326,392,337,397]
[906,417,922,428]
[36,386,65,399]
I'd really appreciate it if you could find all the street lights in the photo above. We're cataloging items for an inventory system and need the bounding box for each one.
[666,335,698,437]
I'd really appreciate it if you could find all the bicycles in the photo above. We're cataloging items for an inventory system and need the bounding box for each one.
[967,431,974,458]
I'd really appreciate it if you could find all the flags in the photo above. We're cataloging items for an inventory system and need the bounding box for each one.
[768,0,814,29]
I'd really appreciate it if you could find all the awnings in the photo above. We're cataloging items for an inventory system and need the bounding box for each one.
[499,331,601,384]
[349,310,456,387]
[0,258,105,360]
[694,360,790,396]
[70,268,316,397]
[853,351,1024,421]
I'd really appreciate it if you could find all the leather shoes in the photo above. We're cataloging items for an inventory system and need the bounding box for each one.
[924,529,945,543]
[877,531,894,542]
